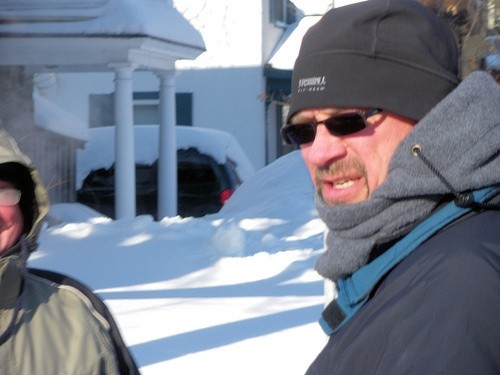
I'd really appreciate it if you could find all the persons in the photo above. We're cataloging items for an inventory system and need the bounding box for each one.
[279,0,500,375]
[0,127,141,375]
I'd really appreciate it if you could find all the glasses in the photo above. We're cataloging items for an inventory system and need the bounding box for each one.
[0,187,24,208]
[278,106,390,146]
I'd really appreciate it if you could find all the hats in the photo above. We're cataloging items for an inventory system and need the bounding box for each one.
[284,0,463,125]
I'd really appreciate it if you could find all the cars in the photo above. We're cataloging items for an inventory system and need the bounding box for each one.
[76,124,240,220]
